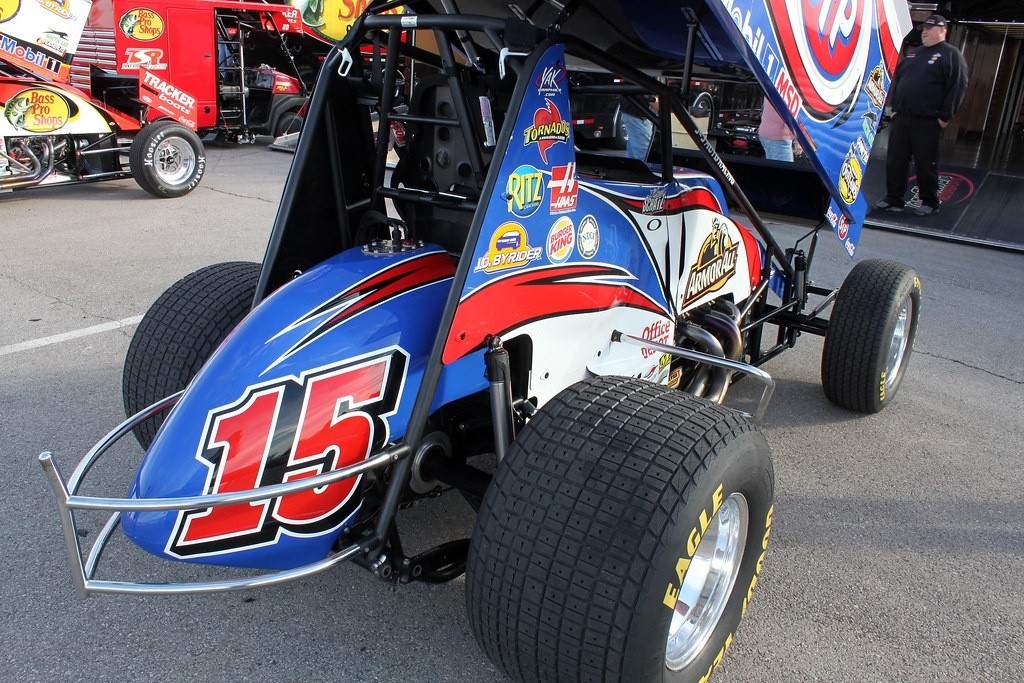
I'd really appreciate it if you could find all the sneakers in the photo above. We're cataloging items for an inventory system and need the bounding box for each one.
[869,199,904,211]
[913,204,939,216]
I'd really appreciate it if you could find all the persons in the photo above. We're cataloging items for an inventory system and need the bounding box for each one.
[759,96,804,162]
[622,82,657,161]
[871,15,969,215]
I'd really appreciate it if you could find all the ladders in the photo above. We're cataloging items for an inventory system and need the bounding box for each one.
[215,14,246,129]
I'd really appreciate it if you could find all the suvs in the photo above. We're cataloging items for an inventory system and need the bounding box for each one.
[1,26,204,200]
[33,0,923,683]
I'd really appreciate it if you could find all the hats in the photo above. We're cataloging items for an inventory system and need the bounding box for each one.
[917,15,948,30]
